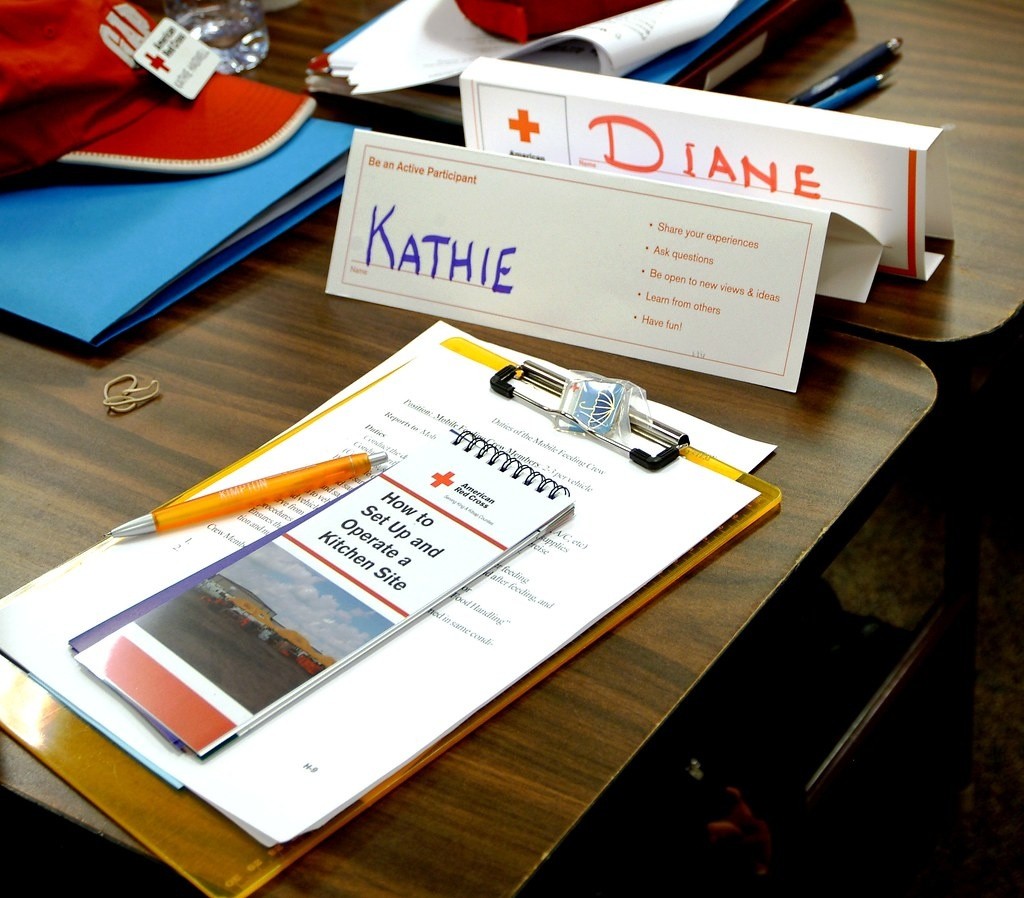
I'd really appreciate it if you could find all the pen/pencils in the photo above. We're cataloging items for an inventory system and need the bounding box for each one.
[102,451,388,540]
[783,35,903,111]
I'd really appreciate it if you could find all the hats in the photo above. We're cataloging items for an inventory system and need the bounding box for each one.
[0,0,316,174]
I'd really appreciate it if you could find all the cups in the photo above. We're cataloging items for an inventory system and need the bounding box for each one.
[160,0,271,76]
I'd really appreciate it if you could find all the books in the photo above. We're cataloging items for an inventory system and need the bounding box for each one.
[70,431,575,761]
